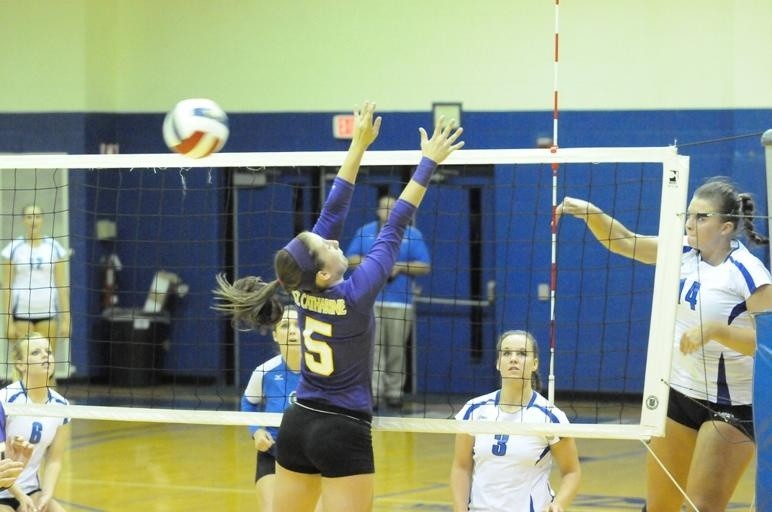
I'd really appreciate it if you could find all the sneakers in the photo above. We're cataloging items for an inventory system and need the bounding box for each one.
[372,394,404,412]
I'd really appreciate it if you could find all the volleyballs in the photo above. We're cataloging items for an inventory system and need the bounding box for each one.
[162,99,230,159]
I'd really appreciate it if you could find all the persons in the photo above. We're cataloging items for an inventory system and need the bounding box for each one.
[209,101,466,512]
[241,304,302,512]
[347,186,430,411]
[552,176,769,512]
[0,204,70,391]
[452,329,582,512]
[0,332,72,512]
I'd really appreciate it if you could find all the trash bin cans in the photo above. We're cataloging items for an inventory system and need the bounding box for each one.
[101,307,170,388]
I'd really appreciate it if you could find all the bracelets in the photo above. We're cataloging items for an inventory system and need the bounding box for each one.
[411,156,438,190]
